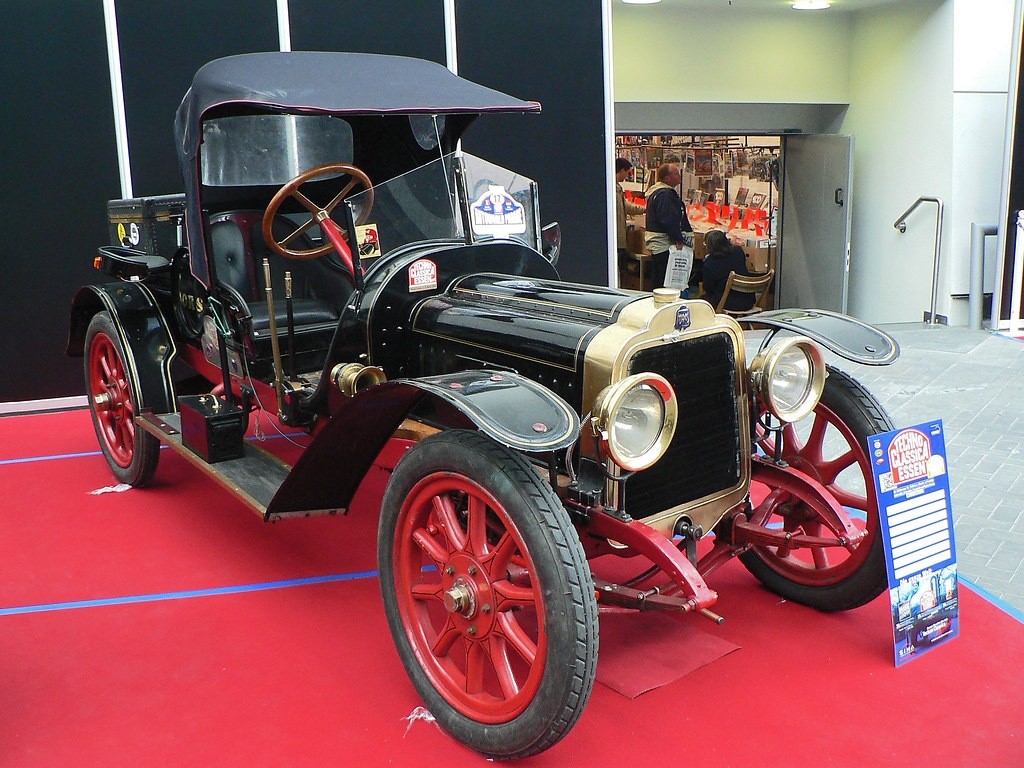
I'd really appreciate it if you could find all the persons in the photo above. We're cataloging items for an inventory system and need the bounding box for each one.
[697,230,756,319]
[615,158,646,264]
[644,164,694,292]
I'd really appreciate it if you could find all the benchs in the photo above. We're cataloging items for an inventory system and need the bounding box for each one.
[204,202,361,379]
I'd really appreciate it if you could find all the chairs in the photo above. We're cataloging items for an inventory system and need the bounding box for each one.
[716,269,776,330]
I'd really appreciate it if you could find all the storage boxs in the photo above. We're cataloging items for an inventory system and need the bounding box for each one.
[617,207,778,269]
